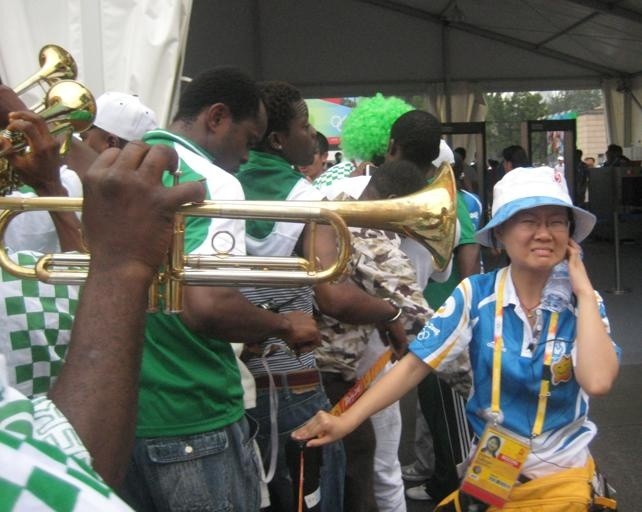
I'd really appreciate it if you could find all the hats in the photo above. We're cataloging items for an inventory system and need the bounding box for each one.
[431,139,456,169]
[471,165,598,248]
[92,90,161,142]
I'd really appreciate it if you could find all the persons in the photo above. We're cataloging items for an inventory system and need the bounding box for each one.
[574,144,631,203]
[0,84,206,512]
[119,68,322,512]
[488,158,500,168]
[298,110,484,512]
[235,83,405,512]
[502,145,528,172]
[291,167,622,512]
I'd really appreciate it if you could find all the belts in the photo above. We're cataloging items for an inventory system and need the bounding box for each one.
[254,367,321,390]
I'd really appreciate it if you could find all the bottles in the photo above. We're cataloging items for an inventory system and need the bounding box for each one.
[541,258,573,312]
[598,153,605,166]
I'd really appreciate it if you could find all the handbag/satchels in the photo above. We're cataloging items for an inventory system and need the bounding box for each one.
[449,452,598,512]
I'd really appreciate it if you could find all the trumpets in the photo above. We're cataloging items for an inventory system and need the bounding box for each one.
[0,81,97,197]
[0,161,457,315]
[9,46,77,113]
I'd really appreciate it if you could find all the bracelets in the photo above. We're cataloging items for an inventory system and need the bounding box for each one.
[382,298,403,323]
